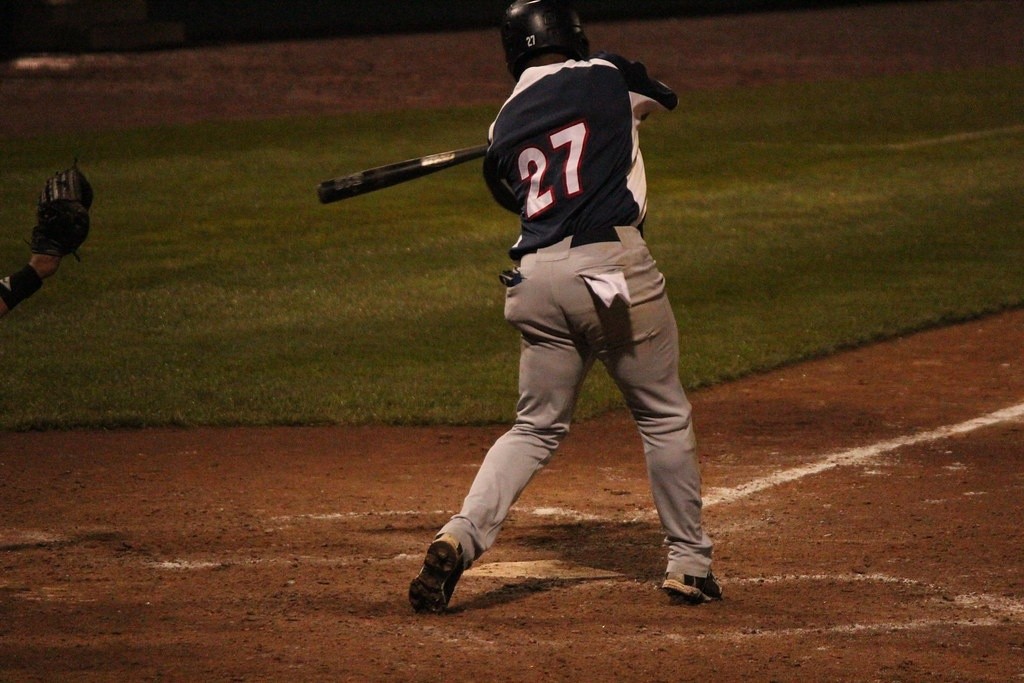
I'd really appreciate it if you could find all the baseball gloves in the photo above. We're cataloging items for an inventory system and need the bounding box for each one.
[28,164,96,259]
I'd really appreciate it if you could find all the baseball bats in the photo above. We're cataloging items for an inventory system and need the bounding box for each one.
[318,142,486,206]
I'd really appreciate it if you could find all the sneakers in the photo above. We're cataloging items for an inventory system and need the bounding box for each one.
[660,572,724,602]
[408,533,465,616]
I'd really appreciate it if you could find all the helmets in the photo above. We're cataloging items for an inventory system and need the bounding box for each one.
[500,0,589,81]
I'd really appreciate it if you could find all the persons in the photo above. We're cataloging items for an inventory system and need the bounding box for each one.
[405,0,725,610]
[0,249,61,315]
